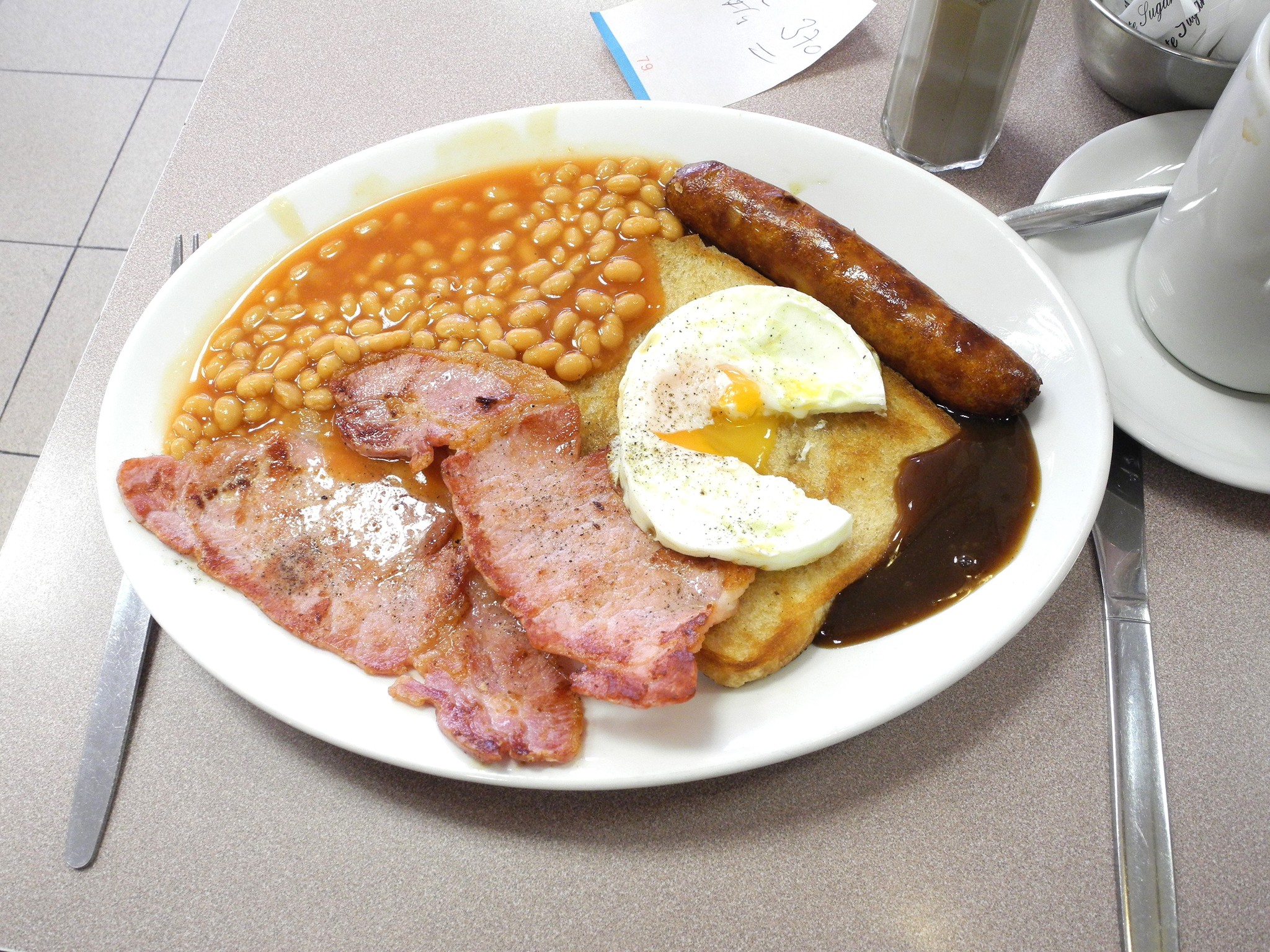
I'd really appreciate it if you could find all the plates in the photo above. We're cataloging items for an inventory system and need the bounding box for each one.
[95,99,1114,791]
[1026,109,1270,493]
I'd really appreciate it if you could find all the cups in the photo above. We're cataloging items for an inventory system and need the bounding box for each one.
[1134,12,1270,394]
[881,0,1043,173]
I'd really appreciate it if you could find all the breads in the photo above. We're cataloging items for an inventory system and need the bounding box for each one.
[555,231,964,689]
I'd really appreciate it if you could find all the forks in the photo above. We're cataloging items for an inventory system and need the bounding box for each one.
[65,234,198,870]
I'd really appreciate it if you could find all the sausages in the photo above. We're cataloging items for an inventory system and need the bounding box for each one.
[665,160,1043,422]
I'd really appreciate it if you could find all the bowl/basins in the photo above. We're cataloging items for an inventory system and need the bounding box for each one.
[1072,0,1240,113]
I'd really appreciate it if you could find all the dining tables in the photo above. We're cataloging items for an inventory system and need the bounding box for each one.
[1,3,1270,952]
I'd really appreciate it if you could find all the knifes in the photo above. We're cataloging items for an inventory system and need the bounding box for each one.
[1091,425,1178,952]
